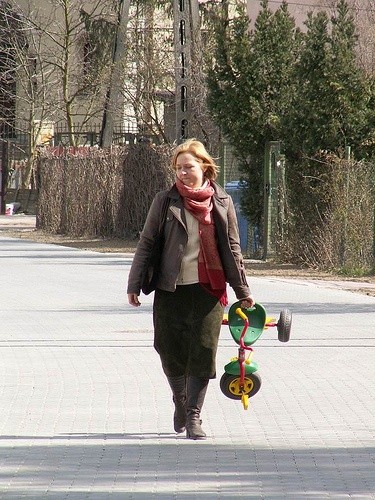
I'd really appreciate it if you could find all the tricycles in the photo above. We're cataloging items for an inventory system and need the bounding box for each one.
[221,297,292,408]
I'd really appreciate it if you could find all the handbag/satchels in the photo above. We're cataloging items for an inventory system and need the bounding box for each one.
[140,197,171,296]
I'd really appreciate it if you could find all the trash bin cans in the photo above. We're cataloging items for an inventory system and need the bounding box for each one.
[224,180,265,260]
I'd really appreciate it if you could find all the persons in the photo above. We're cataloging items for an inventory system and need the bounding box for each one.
[126,140,255,440]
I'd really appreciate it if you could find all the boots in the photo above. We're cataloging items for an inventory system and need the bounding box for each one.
[184,375,209,440]
[167,374,187,433]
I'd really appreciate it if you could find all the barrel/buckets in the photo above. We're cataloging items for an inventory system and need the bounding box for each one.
[6,204,13,215]
